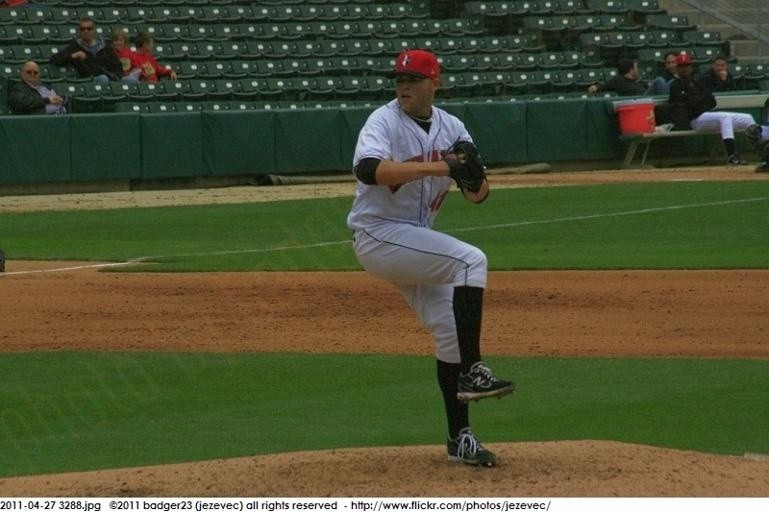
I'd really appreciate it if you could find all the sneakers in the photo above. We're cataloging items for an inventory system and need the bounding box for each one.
[727,155,740,165]
[446,432,494,467]
[456,367,518,403]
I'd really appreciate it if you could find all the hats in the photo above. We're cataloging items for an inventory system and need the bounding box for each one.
[676,55,692,66]
[386,50,439,80]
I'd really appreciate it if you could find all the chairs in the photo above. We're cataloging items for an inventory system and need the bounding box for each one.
[0,1,769,114]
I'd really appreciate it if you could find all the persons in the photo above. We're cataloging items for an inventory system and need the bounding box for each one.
[7,61,71,115]
[347,49,516,468]
[48,17,177,86]
[587,50,769,172]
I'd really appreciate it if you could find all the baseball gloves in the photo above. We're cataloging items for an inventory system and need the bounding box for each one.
[441,136,485,194]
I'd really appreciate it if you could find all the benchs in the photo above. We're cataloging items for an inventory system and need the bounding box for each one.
[604,97,744,167]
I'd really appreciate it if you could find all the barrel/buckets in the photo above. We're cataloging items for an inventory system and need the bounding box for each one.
[616,102,657,134]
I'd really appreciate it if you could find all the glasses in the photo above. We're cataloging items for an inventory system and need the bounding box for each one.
[23,70,39,74]
[77,27,95,33]
